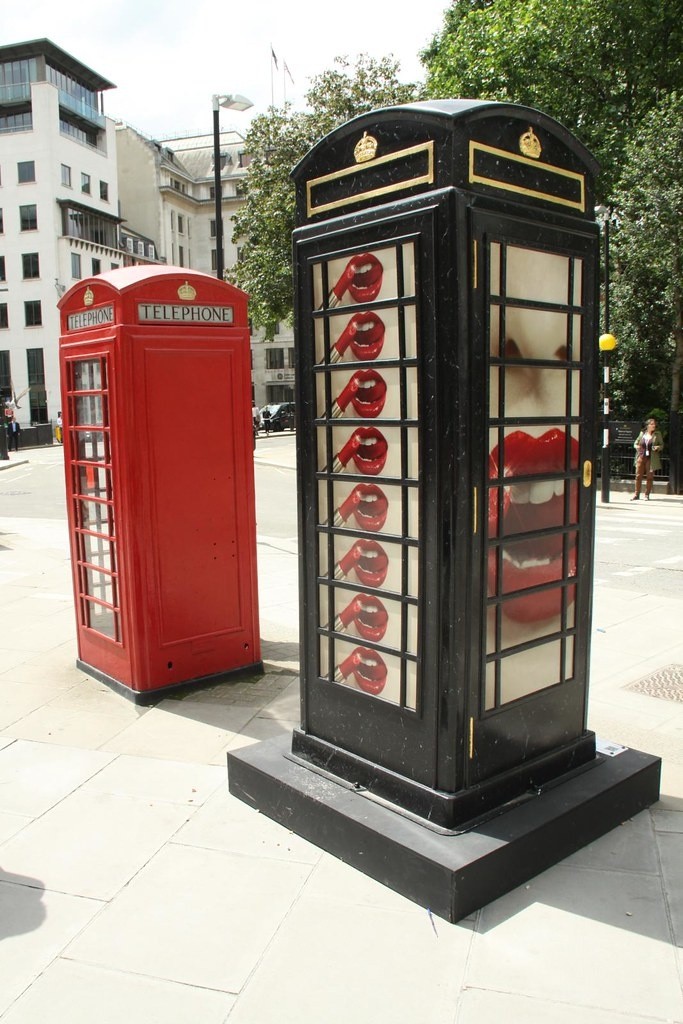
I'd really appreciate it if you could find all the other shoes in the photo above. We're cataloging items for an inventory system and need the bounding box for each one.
[644,496,649,501]
[630,496,640,501]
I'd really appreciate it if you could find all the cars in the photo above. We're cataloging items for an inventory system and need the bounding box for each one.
[255,400,296,432]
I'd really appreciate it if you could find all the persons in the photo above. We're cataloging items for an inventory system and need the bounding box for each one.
[262,406,272,435]
[7,416,20,452]
[57,413,63,443]
[631,419,664,501]
[311,235,584,714]
[252,401,259,436]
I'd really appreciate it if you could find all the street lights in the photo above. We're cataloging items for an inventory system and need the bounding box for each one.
[599,333,617,502]
[211,93,254,282]
